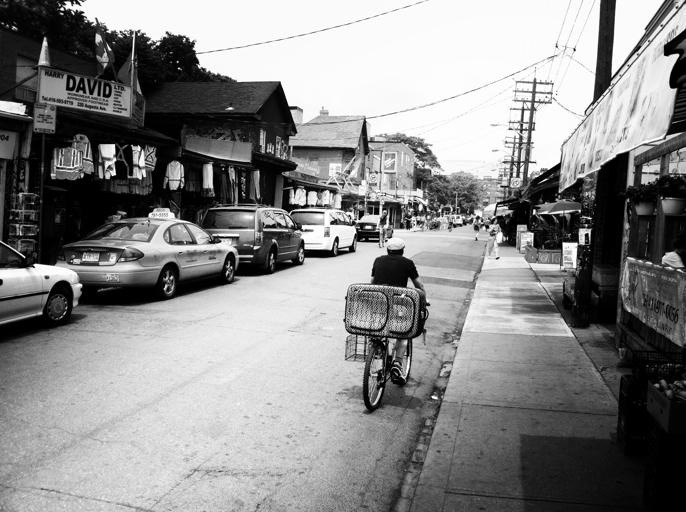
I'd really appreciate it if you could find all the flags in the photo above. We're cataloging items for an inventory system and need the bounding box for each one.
[92,22,116,81]
[115,47,144,96]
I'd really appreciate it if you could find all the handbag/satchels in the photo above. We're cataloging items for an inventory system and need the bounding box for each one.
[496,232,503,243]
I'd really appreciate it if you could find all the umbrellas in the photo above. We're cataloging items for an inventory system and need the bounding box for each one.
[485,214,504,229]
[535,197,583,238]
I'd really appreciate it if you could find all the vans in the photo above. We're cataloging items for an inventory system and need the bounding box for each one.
[443,215,463,225]
[289,208,358,256]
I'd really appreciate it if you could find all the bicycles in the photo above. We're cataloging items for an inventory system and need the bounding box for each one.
[362,302,430,410]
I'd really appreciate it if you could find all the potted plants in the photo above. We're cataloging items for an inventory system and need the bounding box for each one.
[618,174,686,217]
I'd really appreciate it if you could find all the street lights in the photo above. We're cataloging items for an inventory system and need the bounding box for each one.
[491,122,533,195]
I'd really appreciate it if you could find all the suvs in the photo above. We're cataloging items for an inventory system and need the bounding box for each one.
[199,203,305,273]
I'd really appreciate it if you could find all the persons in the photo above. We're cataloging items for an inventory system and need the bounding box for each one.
[345,207,355,221]
[659,237,686,272]
[484,215,502,259]
[401,207,492,243]
[366,235,427,384]
[375,210,390,250]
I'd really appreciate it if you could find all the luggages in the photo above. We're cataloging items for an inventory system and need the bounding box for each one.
[343,283,426,339]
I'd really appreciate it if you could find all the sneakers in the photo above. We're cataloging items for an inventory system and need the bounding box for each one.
[390,360,406,385]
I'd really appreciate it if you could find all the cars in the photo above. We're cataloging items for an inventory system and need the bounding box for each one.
[356,213,393,242]
[53,207,239,299]
[0,239,83,325]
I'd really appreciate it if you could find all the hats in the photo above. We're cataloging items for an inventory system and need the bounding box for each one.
[387,238,405,250]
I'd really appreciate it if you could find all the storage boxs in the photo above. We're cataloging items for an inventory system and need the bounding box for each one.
[591,265,620,287]
[646,378,686,434]
